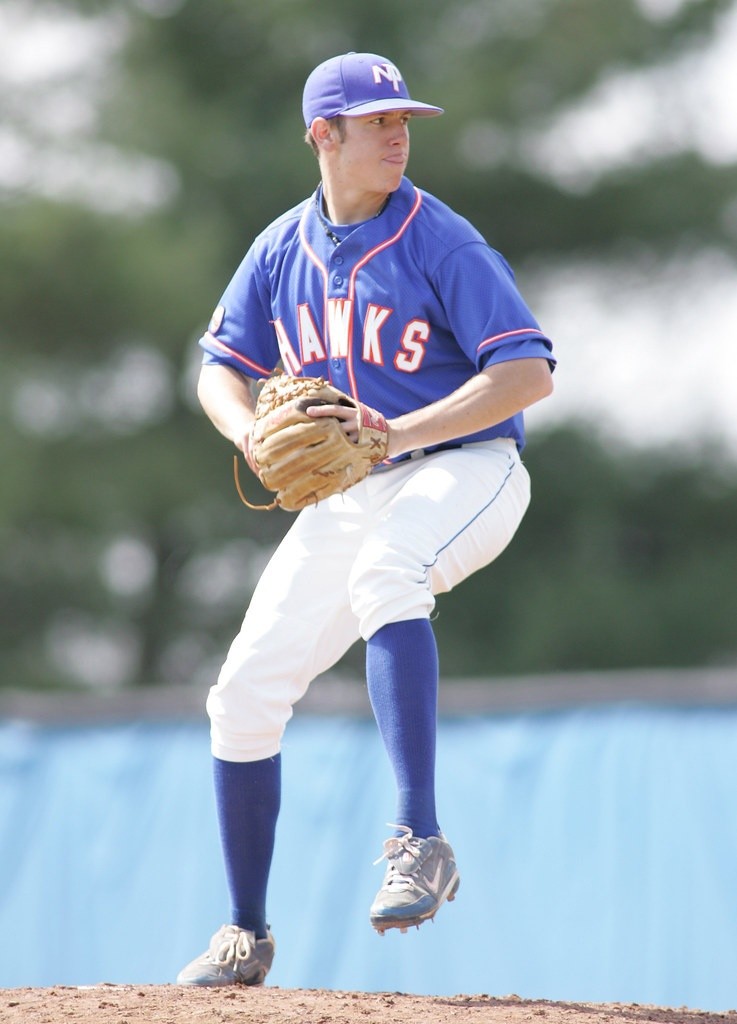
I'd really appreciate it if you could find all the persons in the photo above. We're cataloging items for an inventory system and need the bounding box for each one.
[175,51,556,989]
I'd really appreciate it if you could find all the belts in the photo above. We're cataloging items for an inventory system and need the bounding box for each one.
[396,442,462,462]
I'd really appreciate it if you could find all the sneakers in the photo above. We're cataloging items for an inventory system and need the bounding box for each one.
[176,922,275,987]
[370,821,460,935]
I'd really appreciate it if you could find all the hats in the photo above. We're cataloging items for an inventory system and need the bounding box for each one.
[303,51,444,129]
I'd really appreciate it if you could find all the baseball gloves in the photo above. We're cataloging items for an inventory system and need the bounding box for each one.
[247,373,390,512]
